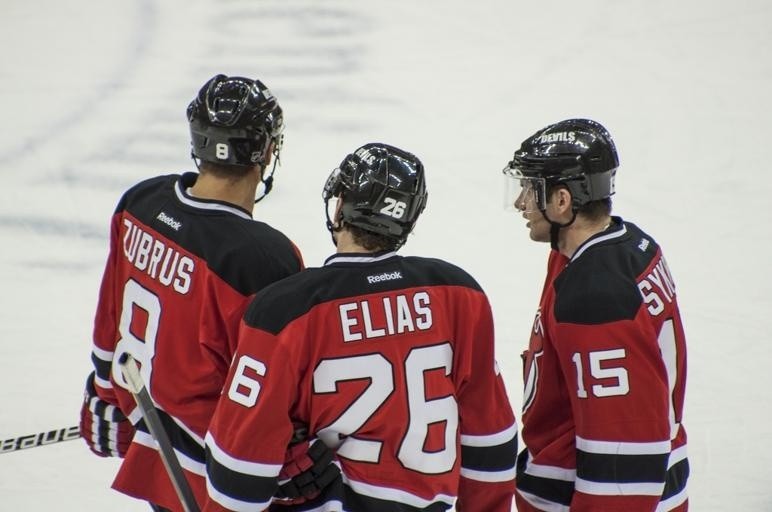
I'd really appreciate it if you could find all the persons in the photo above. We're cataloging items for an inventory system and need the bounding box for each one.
[80,74,305,511]
[504,119,689,512]
[204,145,518,512]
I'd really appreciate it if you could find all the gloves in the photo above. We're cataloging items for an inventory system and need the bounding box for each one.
[79,369,134,459]
[270,438,343,511]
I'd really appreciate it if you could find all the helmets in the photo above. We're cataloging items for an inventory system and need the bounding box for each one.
[503,118,620,213]
[186,73,295,167]
[322,142,429,245]
